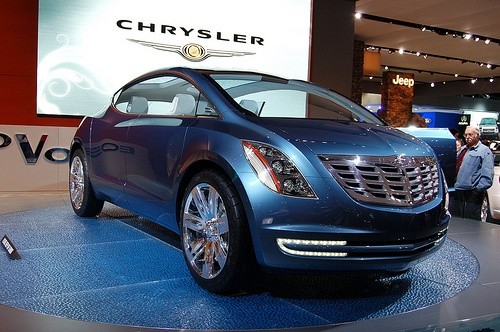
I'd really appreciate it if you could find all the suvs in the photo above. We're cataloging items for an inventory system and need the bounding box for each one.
[477,118,500,141]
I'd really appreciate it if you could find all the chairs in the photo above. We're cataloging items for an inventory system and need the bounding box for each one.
[126,96,148,114]
[240,99,258,114]
[170,93,196,116]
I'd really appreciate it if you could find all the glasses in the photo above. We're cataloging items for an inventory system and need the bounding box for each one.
[463,133,476,137]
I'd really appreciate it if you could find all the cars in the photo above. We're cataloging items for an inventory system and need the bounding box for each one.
[67,66,456,293]
[480,141,500,224]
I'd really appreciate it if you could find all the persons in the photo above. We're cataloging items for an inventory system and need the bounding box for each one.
[455,125,494,221]
[454,133,490,181]
